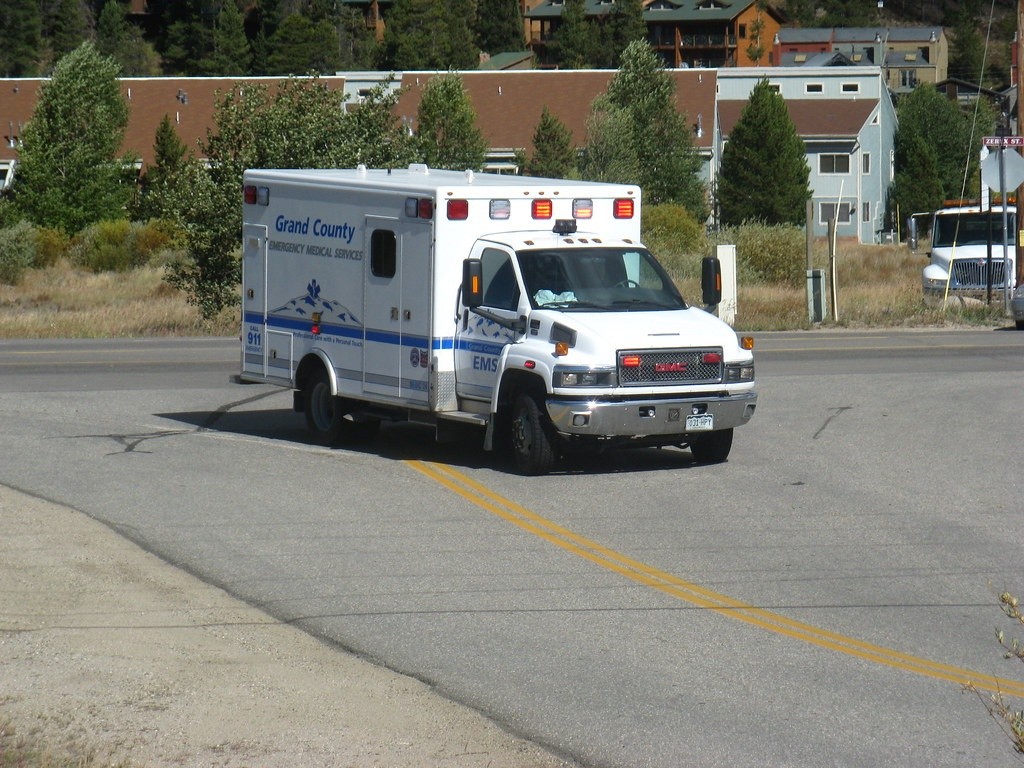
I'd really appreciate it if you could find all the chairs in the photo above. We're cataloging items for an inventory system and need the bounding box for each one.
[580,257,611,288]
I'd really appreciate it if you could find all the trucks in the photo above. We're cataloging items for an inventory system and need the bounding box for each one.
[241,164,757,476]
[906,206,1016,296]
